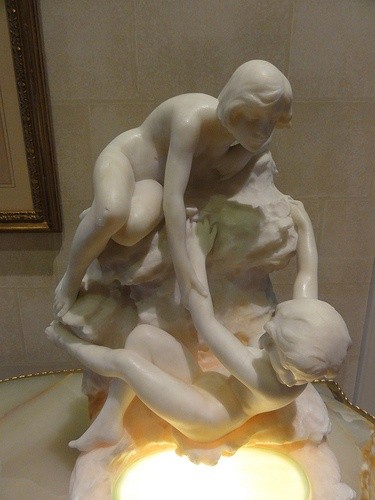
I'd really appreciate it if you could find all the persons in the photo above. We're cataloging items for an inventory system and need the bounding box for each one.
[43,192,351,452]
[52,59,295,319]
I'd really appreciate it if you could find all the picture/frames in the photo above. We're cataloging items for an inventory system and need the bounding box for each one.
[1,1,65,234]
